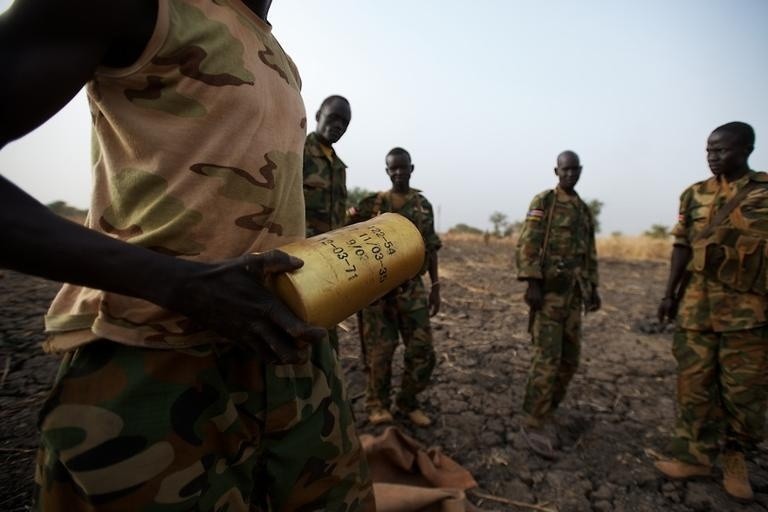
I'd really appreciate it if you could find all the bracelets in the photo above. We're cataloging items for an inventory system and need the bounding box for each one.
[431,281,439,288]
[663,292,675,301]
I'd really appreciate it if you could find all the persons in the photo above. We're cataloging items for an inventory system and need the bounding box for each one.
[659,121,767,501]
[356,148,441,427]
[515,151,601,428]
[1,0,375,510]
[304,98,350,354]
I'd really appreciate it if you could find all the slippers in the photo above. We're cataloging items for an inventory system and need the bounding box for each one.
[520,423,579,459]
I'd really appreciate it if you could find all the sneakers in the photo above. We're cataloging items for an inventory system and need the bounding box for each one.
[408,410,431,427]
[655,460,710,479]
[368,407,393,425]
[719,452,754,501]
